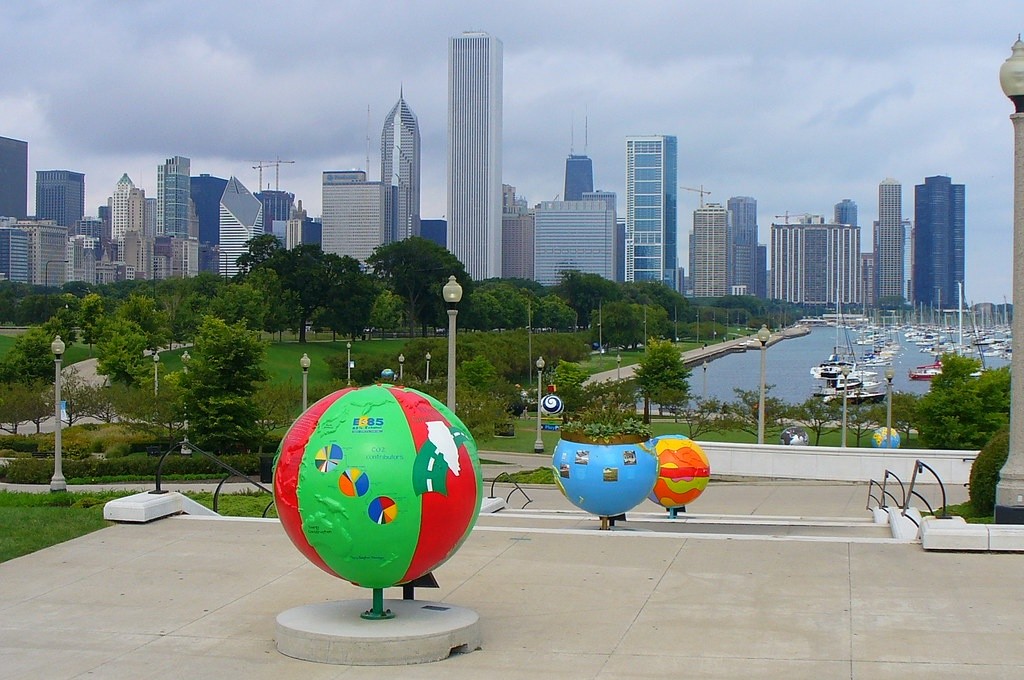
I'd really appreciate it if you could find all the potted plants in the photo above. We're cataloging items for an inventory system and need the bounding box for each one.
[551,392,660,517]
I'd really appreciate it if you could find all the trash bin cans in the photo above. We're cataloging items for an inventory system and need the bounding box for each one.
[146,446,161,456]
[260,457,274,483]
[381,368,395,380]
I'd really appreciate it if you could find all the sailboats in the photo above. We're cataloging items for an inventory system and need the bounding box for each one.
[810,281,1012,404]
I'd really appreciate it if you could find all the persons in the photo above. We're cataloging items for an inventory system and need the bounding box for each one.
[394,371,399,381]
[513,383,531,420]
[616,354,621,369]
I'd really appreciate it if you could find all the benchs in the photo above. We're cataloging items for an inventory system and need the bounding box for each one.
[32,452,67,458]
[259,457,273,483]
[146,446,161,456]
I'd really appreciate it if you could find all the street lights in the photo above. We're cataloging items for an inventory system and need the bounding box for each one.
[616,353,622,376]
[346,342,352,381]
[181,351,193,457]
[399,353,405,381]
[426,352,431,381]
[535,355,545,452]
[757,325,769,444]
[884,367,894,447]
[46,260,70,298]
[441,274,463,416]
[153,353,159,394]
[203,250,229,284]
[300,354,310,412]
[702,359,707,399]
[153,267,179,302]
[48,335,69,493]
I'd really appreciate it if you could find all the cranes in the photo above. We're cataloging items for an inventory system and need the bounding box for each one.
[678,185,711,208]
[252,158,294,192]
[775,211,808,224]
